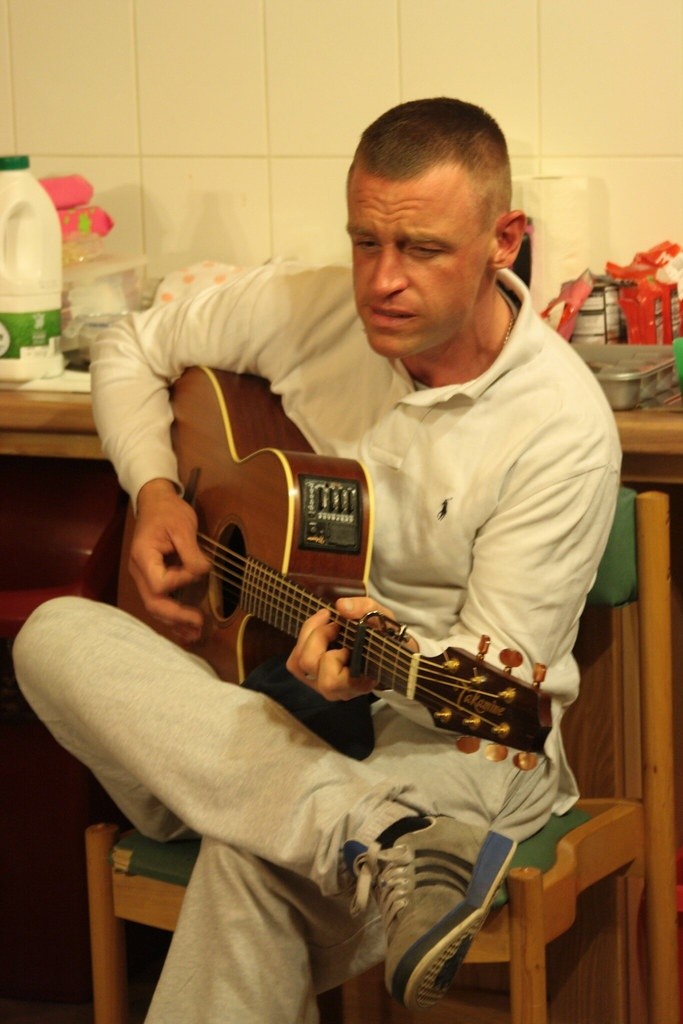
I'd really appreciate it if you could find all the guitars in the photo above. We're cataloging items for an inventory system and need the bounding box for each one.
[113,362,558,774]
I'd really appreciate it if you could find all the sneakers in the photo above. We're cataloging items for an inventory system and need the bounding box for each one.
[341,814,519,1010]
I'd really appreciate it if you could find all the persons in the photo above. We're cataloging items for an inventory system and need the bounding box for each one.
[12,97,622,1024]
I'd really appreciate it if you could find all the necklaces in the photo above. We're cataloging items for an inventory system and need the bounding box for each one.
[465,315,514,383]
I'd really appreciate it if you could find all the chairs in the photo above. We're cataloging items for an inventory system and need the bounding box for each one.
[84,489,683,1024]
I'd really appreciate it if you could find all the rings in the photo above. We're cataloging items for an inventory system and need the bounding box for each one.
[306,673,315,680]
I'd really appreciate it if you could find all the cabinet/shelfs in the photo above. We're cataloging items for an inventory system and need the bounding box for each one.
[0,358,682,641]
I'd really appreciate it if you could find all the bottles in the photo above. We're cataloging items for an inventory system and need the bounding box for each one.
[0,154,68,379]
